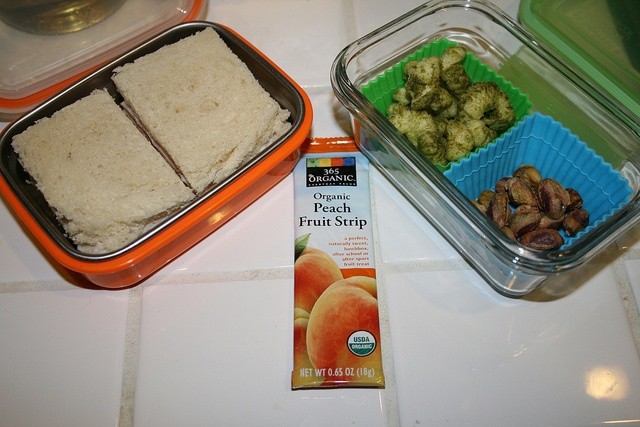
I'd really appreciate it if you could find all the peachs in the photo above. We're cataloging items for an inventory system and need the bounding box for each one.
[294,247,348,313]
[310,276,383,385]
[292,308,326,388]
